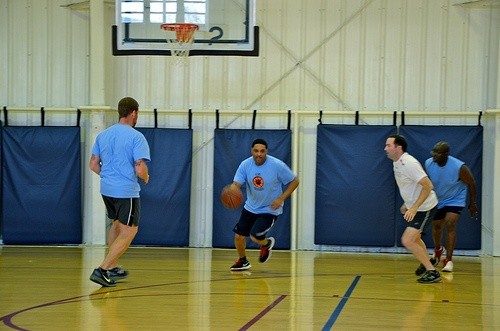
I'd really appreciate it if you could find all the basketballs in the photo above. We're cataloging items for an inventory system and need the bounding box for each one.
[221,184,244,208]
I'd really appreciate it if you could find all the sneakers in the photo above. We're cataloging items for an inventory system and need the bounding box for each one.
[107,266,129,278]
[441,256,453,272]
[433,246,445,265]
[259,237,275,263]
[230,261,251,270]
[417,269,442,284]
[416,258,436,276]
[90,268,115,286]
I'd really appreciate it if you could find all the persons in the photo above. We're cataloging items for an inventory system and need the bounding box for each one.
[423,140,479,271]
[384,134,442,283]
[89,97,151,287]
[230,139,299,271]
